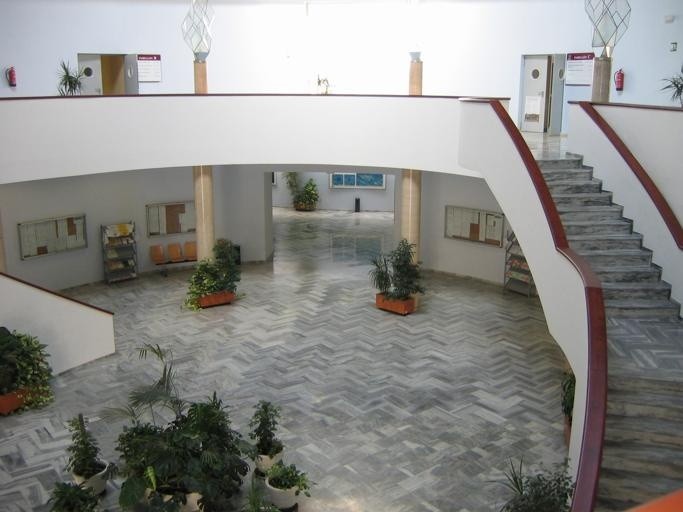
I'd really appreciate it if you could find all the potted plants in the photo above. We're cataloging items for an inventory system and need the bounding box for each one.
[282,170,320,213]
[0,324,51,417]
[178,238,244,314]
[362,236,422,318]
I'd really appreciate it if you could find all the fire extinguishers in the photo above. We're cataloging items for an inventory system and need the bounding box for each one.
[614,68,624,91]
[5,66,16,86]
